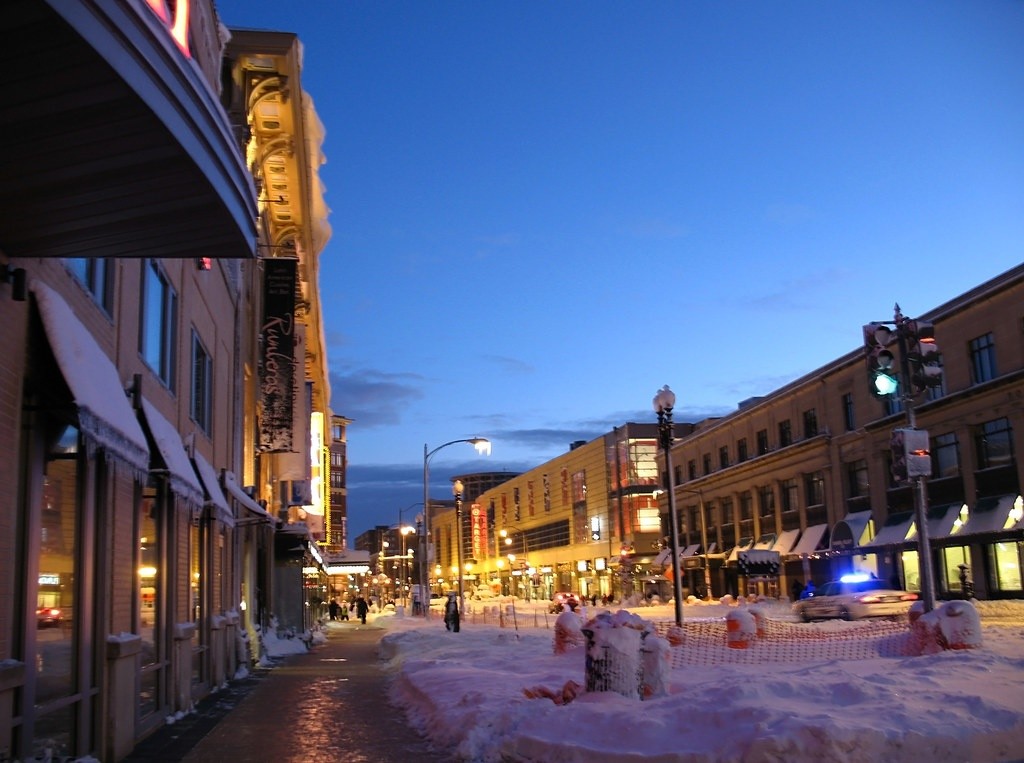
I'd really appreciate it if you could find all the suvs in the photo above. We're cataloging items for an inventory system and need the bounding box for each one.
[548,593,581,614]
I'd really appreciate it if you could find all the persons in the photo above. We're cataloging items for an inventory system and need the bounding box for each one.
[581,594,615,607]
[328,597,369,624]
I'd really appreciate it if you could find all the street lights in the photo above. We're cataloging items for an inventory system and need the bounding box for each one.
[424,436,491,611]
[502,524,531,602]
[654,487,713,602]
[380,524,410,610]
[415,512,423,614]
[453,478,466,622]
[652,385,684,629]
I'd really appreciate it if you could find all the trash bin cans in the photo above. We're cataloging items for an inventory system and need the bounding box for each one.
[578,613,667,701]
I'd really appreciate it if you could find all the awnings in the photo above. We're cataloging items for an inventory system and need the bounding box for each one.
[770,529,799,555]
[728,538,753,561]
[906,501,964,541]
[663,547,685,564]
[865,511,915,546]
[223,479,276,527]
[843,511,872,547]
[139,394,205,505]
[952,495,1018,536]
[194,449,241,527]
[679,544,700,562]
[793,524,827,553]
[28,282,150,473]
[753,533,774,550]
[653,549,672,565]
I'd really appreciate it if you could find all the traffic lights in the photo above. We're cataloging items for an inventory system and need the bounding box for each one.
[907,320,943,388]
[862,323,900,399]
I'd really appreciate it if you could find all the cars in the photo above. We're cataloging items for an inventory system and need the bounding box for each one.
[791,576,918,621]
